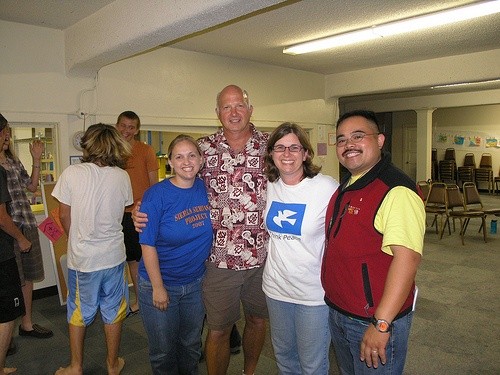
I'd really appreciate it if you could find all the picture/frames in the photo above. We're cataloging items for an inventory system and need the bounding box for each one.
[70,156,82,164]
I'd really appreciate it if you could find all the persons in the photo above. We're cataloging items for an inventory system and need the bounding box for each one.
[111,109,159,315]
[50,123,134,375]
[0,112,45,375]
[130,84,426,375]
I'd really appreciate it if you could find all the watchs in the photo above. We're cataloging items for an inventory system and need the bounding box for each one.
[371,314,392,333]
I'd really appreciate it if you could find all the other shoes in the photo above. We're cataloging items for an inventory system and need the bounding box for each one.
[230,335,241,353]
[19,323,54,338]
[7,344,16,355]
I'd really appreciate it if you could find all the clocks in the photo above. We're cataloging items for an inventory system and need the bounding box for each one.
[73,131,87,150]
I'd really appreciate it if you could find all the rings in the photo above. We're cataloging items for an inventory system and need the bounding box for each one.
[372,351,378,353]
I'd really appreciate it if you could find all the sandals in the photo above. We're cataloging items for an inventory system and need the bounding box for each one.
[126,306,140,319]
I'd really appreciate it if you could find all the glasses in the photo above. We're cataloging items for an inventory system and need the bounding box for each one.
[337,134,379,145]
[271,145,304,152]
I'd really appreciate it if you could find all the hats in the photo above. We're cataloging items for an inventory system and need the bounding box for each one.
[0,113,8,132]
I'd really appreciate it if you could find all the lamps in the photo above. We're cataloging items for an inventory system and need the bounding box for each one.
[282,0,500,56]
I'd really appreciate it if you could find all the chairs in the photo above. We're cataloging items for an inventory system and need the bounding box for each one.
[417,148,500,245]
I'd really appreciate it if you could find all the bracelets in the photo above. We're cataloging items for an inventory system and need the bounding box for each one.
[32,165,40,169]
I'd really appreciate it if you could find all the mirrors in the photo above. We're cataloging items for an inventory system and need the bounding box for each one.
[8,122,60,215]
